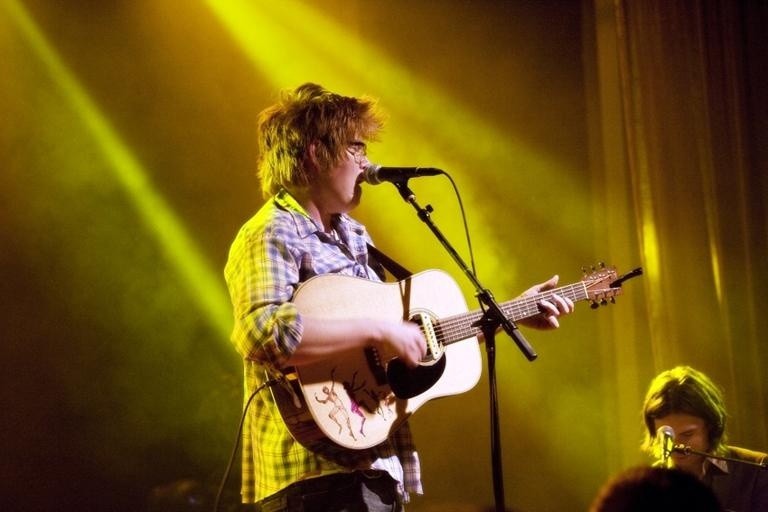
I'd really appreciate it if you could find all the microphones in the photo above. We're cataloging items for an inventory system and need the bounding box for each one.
[362,163,441,185]
[656,424,676,460]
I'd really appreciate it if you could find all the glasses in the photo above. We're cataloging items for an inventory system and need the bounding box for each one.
[348,141,367,154]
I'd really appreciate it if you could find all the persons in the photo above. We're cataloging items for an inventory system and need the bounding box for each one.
[222,80,575,512]
[588,463,723,512]
[639,364,768,512]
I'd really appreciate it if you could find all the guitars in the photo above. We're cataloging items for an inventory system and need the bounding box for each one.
[263,261,641,456]
